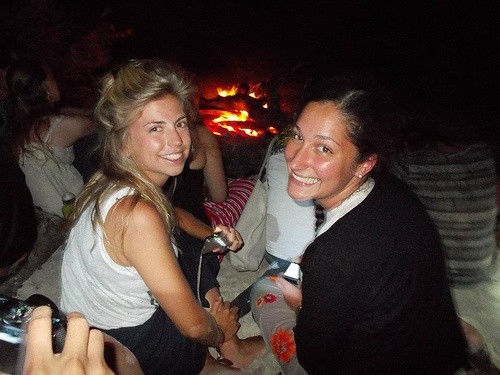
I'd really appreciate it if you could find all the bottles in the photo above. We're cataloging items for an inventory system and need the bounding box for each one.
[62,192,76,218]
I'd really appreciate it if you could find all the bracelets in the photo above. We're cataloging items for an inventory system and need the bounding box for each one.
[212,325,225,358]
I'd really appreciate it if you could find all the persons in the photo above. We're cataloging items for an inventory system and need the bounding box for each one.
[160,99,227,279]
[60,58,266,375]
[250,84,489,375]
[22,305,144,375]
[264,112,317,277]
[0,61,99,224]
[387,118,498,286]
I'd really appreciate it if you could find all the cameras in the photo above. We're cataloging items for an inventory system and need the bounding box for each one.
[0,293,67,375]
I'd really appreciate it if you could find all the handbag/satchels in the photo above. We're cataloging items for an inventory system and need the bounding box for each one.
[226,134,279,271]
